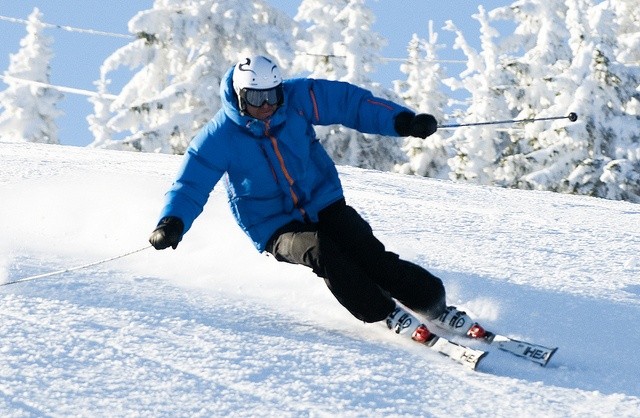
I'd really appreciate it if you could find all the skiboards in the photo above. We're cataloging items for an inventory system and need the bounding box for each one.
[409,322,558,370]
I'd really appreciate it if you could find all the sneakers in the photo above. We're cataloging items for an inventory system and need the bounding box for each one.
[428,308,472,335]
[385,307,421,340]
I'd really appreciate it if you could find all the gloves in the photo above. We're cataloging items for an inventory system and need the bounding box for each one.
[149,217,184,250]
[396,112,438,139]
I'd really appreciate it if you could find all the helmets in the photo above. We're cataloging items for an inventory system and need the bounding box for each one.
[231,50,284,108]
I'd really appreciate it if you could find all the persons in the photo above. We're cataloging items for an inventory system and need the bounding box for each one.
[149,55,485,342]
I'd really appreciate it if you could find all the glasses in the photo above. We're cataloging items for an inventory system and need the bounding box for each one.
[240,88,282,107]
